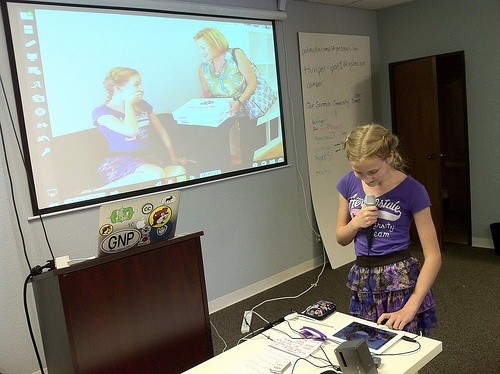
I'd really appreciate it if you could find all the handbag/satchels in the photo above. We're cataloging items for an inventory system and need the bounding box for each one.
[232,47,277,121]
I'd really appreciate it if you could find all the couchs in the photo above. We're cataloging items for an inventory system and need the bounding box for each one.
[52,107,230,195]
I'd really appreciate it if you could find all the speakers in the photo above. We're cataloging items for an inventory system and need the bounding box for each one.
[334,338,378,374]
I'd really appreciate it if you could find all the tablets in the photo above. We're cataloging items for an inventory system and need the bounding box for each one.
[326,319,405,355]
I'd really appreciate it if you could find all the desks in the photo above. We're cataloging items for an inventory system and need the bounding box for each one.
[181,314,443,374]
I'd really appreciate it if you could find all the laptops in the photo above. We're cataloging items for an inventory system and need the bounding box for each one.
[95,191,181,258]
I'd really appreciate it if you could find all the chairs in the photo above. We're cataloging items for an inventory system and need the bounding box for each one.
[253,62,278,158]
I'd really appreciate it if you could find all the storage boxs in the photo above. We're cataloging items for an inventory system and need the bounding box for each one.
[172,97,233,127]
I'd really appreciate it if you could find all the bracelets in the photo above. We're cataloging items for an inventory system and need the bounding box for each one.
[238,99,243,105]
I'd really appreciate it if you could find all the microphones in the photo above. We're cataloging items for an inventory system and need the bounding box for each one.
[363,194,377,249]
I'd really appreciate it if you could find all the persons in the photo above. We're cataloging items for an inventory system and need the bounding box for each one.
[193,27,258,167]
[335,124,442,337]
[79,67,186,194]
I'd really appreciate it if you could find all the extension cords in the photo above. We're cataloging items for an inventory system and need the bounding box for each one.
[241,311,252,333]
